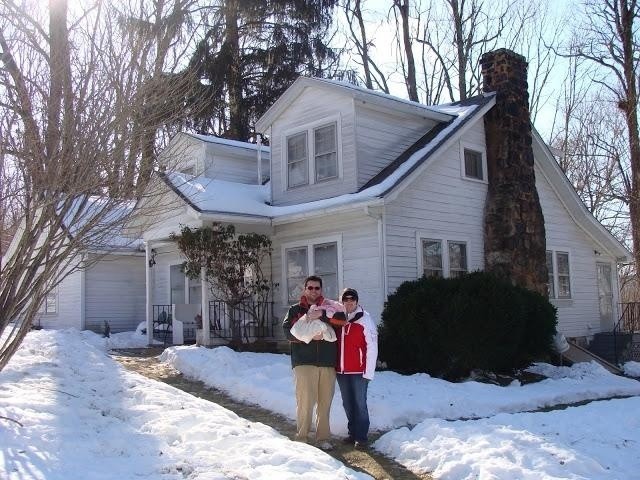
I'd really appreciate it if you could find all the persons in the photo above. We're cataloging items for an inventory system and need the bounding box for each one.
[334,287,380,448]
[282,275,346,450]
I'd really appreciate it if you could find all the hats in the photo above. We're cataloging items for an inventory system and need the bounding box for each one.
[341,288,358,300]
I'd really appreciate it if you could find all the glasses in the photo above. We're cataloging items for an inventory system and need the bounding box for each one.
[342,296,356,302]
[306,286,322,291]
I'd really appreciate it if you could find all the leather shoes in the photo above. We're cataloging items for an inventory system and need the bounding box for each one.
[343,436,354,442]
[354,440,368,449]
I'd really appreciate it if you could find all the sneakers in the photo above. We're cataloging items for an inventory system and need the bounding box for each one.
[315,441,333,450]
